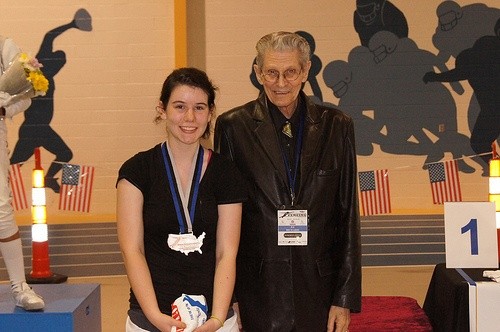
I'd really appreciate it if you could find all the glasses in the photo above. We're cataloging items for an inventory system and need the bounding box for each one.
[260,65,303,83]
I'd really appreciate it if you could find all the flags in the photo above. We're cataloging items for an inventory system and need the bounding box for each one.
[427,159,463,205]
[358,169,392,215]
[57,162,95,214]
[6,164,29,212]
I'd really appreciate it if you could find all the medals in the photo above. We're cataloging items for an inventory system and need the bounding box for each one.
[166,231,207,257]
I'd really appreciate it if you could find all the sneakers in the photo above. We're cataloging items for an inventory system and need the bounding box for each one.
[13,282,45,310]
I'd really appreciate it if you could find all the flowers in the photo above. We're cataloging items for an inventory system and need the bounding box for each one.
[0,49,52,104]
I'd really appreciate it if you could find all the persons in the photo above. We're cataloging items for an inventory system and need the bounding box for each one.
[213,30,364,332]
[1,33,49,313]
[114,66,245,332]
